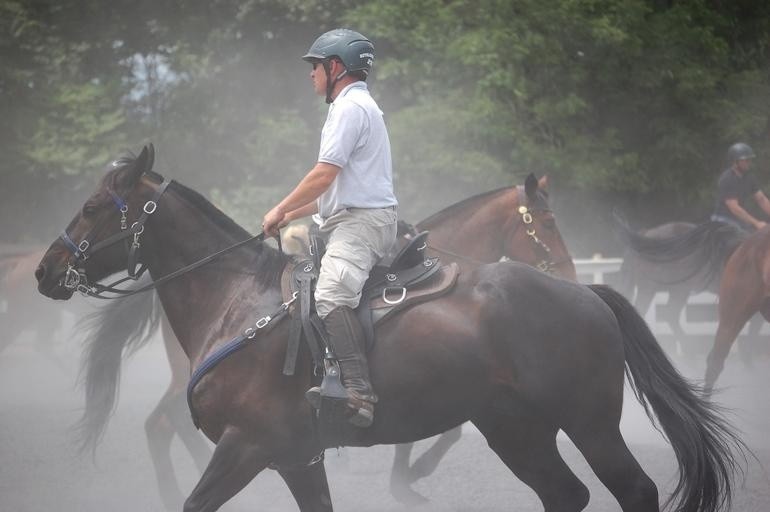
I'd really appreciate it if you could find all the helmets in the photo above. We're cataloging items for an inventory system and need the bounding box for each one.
[727,144,756,161]
[302,29,375,81]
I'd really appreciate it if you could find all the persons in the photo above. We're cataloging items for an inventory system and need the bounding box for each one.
[262,29,397,429]
[710,143,769,236]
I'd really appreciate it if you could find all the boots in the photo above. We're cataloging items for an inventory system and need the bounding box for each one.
[306,306,378,428]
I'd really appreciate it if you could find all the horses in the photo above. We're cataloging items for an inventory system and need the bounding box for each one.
[72,169,585,511]
[31,139,762,512]
[609,214,770,404]
[0,239,54,350]
[608,218,737,371]
